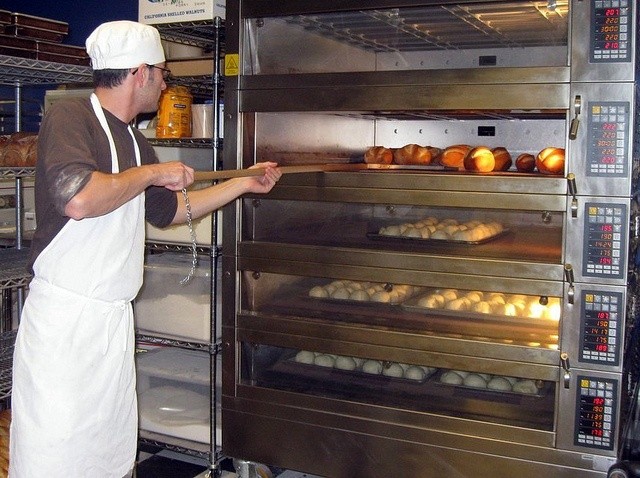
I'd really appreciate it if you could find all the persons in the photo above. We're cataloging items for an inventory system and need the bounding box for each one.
[7,18,284,478]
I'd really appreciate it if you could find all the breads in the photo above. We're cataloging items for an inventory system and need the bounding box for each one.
[465,375,489,388]
[340,355,353,372]
[505,373,518,386]
[472,302,496,314]
[367,214,505,242]
[427,368,438,376]
[1,399,14,477]
[440,290,456,300]
[532,299,545,316]
[318,355,337,368]
[505,295,531,315]
[544,297,561,319]
[467,146,495,174]
[296,350,316,365]
[536,145,563,173]
[467,291,485,301]
[518,379,538,397]
[362,361,383,374]
[492,375,510,390]
[419,294,440,310]
[407,366,425,381]
[400,143,433,168]
[384,363,404,376]
[441,141,474,167]
[445,296,469,312]
[305,279,417,312]
[1,129,45,167]
[492,145,512,168]
[425,141,443,153]
[487,295,505,305]
[363,145,396,162]
[516,148,532,169]
[442,372,460,384]
[458,371,469,377]
[354,360,363,370]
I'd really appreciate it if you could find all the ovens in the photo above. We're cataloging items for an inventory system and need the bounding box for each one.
[209,1,640,477]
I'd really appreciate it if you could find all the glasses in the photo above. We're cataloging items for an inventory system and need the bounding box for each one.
[132,64,171,76]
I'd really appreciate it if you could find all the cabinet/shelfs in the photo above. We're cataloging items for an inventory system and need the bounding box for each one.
[0,55,95,400]
[129,16,228,478]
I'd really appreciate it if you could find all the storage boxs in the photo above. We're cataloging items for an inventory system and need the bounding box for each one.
[137,0,228,25]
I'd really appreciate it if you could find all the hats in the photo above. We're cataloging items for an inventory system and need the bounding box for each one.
[85,19,165,70]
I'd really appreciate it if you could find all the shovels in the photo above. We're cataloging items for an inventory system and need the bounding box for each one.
[194,162,446,181]
